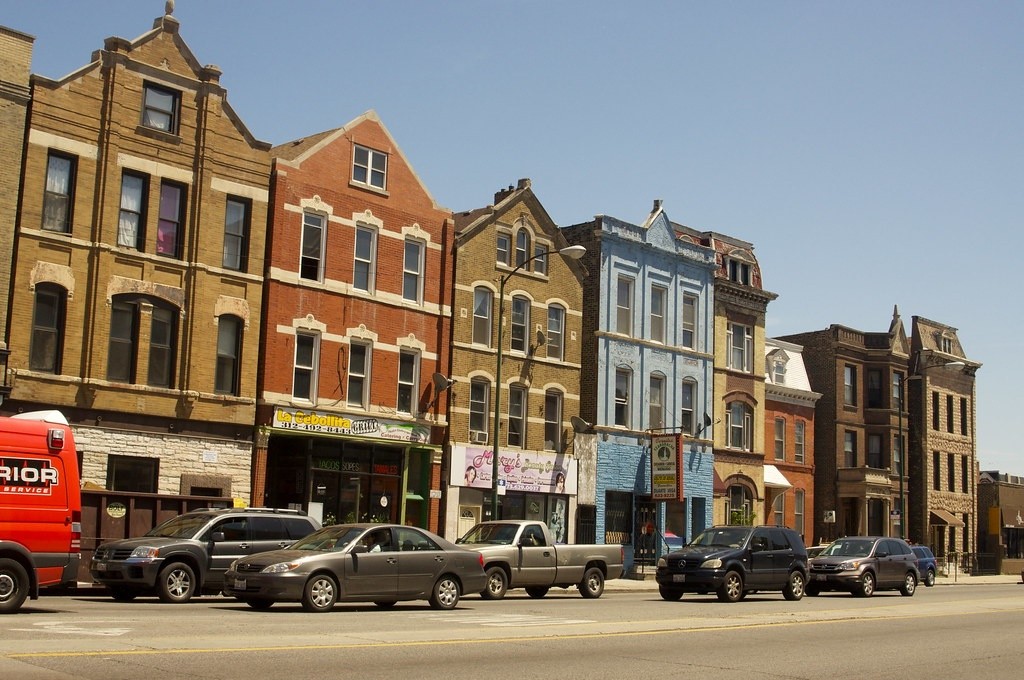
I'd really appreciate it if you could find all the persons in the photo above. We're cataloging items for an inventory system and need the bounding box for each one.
[553,472,567,493]
[360,533,384,552]
[464,466,477,486]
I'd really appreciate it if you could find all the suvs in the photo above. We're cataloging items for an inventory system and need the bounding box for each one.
[88,506,333,604]
[654,524,811,604]
[804,536,920,598]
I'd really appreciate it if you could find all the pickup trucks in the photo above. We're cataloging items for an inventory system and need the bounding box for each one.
[454,520,627,600]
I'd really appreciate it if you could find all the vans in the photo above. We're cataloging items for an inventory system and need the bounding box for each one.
[0,409,83,615]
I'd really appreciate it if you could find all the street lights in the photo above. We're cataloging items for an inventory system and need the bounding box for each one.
[898,361,965,541]
[490,243,587,521]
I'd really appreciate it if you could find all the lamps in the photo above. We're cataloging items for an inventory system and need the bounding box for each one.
[602,432,608,441]
[530,331,546,355]
[637,435,647,445]
[424,373,458,412]
[701,443,707,452]
[697,411,721,435]
[564,416,592,446]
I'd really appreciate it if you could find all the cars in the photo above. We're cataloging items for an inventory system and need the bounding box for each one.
[221,524,488,614]
[910,544,939,587]
[806,543,865,562]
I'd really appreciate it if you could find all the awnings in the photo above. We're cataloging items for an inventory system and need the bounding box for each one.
[932,510,966,528]
[761,463,792,489]
